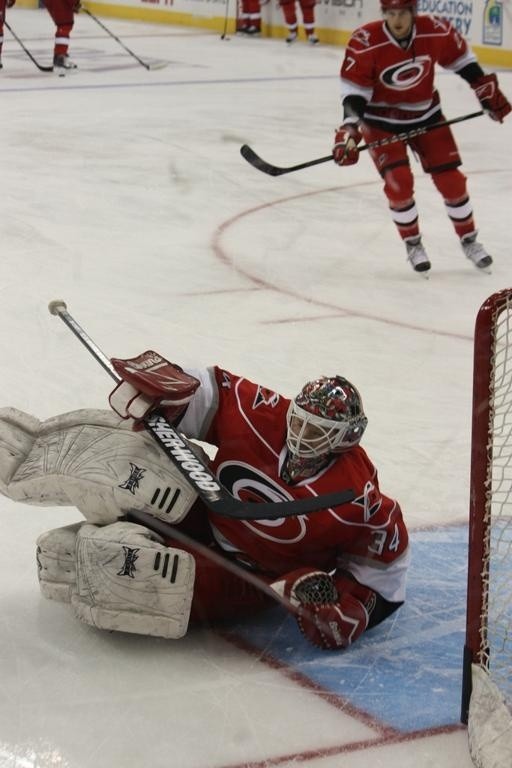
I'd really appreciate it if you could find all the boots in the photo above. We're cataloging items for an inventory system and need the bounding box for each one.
[285,32,319,44]
[400,233,431,272]
[53,51,78,70]
[457,232,493,269]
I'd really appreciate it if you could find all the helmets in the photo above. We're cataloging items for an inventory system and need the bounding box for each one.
[376,0,418,12]
[283,374,369,461]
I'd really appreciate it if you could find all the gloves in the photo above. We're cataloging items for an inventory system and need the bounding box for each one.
[333,123,361,167]
[285,563,371,653]
[471,72,512,123]
[105,348,200,435]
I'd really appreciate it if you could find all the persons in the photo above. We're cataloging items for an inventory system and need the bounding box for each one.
[234,0,264,36]
[1,346,413,654]
[41,0,83,71]
[330,0,511,276]
[278,0,321,47]
[0,0,20,70]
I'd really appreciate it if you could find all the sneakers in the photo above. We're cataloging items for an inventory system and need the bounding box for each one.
[236,24,261,36]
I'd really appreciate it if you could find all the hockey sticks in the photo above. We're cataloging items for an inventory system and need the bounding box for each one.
[49,299,356,519]
[241,108,490,177]
[77,5,170,70]
[4,24,55,73]
[220,0,232,42]
